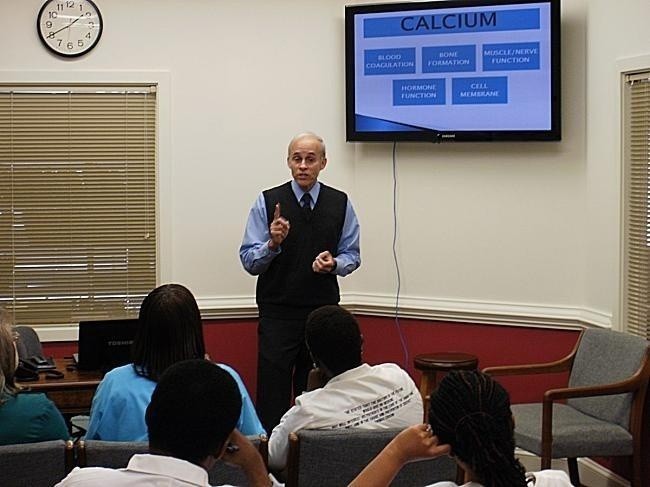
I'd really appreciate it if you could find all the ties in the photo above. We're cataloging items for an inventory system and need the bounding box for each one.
[301,192,313,223]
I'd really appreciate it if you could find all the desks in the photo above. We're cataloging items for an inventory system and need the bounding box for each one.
[15,357,103,439]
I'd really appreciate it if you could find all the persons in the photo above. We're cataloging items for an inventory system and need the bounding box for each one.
[0,306,71,445]
[84,281,267,440]
[237,129,362,428]
[52,356,288,487]
[267,304,426,471]
[343,366,577,486]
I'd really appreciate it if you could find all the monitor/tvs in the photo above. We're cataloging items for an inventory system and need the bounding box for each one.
[345,0,561,141]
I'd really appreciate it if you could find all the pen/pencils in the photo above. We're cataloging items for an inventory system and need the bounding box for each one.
[227,442,240,453]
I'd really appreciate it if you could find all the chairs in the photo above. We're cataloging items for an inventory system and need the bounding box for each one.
[0,439,75,487]
[283,426,463,487]
[11,325,45,358]
[463,326,650,487]
[76,433,268,487]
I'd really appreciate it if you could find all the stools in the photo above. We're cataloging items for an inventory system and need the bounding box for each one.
[412,352,480,424]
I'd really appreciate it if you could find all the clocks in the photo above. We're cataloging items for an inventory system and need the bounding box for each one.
[36,0,104,58]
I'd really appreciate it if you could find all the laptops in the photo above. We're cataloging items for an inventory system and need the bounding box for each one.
[72,319,139,370]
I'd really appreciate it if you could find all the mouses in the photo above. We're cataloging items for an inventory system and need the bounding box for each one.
[32,354,48,365]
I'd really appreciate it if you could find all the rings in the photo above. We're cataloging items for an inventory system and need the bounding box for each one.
[426,423,434,434]
